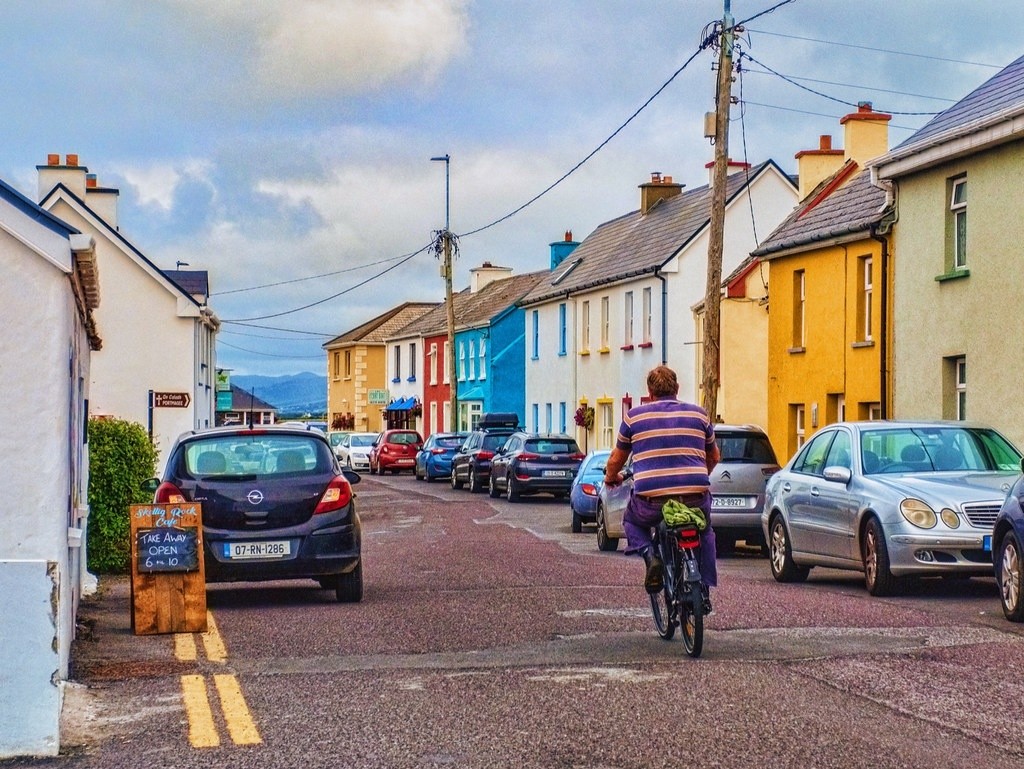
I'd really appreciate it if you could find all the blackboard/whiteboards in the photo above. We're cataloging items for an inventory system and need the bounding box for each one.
[138,526,199,572]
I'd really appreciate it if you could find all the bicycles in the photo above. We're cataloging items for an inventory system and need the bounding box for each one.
[610,468,710,657]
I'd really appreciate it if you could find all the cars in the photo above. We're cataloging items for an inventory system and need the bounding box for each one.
[592,424,784,551]
[367,429,424,475]
[760,419,1024,596]
[569,448,616,531]
[139,421,365,603]
[991,456,1024,623]
[413,430,472,483]
[230,419,380,471]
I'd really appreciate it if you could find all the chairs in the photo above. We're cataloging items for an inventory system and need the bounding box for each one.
[932,447,963,470]
[818,448,851,475]
[900,444,937,472]
[273,451,306,473]
[849,451,879,474]
[405,436,414,443]
[196,451,226,473]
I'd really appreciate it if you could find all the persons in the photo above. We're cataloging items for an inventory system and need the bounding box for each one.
[605,367,720,613]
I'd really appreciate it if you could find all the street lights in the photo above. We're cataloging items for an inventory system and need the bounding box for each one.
[430,154,460,436]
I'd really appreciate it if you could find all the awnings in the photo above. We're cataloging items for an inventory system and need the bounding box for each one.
[385,397,413,411]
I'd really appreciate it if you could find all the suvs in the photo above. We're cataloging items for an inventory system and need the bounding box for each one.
[450,412,527,492]
[487,429,586,503]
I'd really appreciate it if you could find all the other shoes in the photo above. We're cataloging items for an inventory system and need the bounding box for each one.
[699,587,712,615]
[643,546,666,596]
